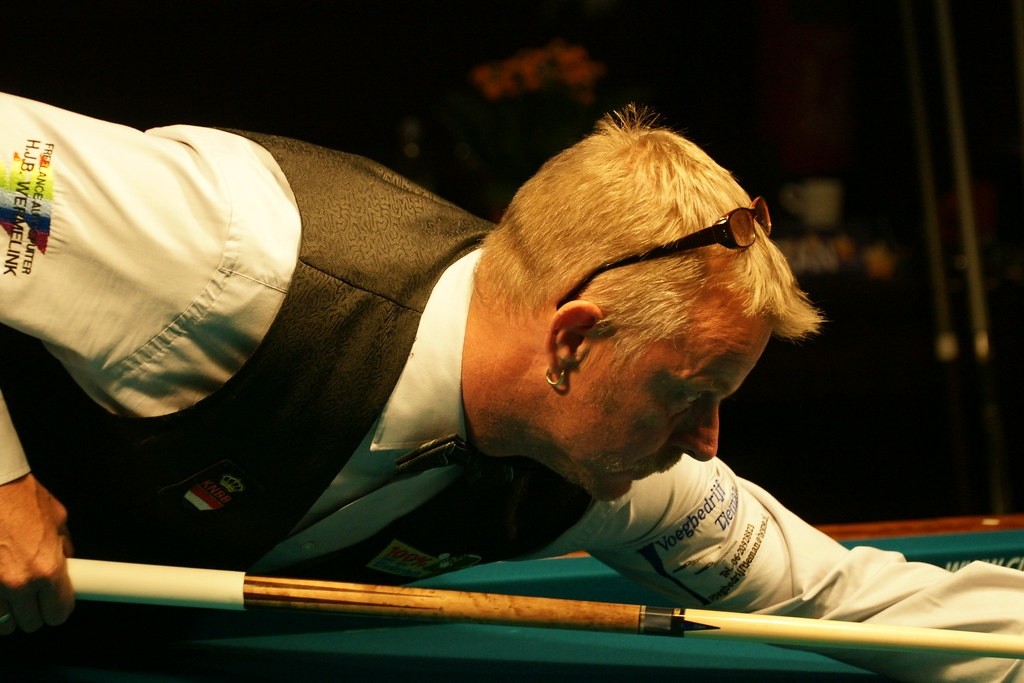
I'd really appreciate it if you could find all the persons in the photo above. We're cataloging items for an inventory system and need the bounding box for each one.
[0,88,1024,683]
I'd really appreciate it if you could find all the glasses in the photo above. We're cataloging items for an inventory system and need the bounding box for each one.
[556,195,771,311]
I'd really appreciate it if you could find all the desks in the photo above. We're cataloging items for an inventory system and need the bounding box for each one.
[0,513,1024,683]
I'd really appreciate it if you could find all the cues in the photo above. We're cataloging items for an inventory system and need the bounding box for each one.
[64,555,1024,659]
